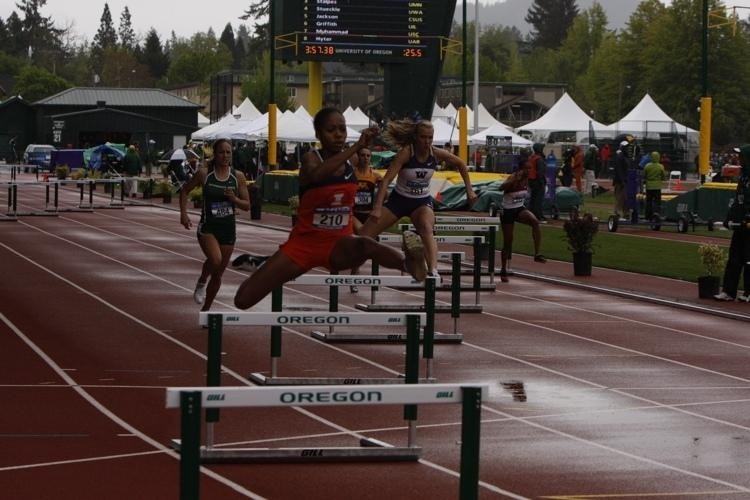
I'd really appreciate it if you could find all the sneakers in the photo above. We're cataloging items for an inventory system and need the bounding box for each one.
[229,251,271,273]
[618,217,627,222]
[401,230,429,284]
[533,252,548,265]
[538,219,548,224]
[132,192,136,198]
[193,279,207,305]
[125,192,130,198]
[713,291,736,302]
[428,269,443,286]
[642,219,651,223]
[654,212,661,222]
[737,293,750,303]
[499,269,510,284]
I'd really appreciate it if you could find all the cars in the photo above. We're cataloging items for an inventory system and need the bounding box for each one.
[23,144,58,174]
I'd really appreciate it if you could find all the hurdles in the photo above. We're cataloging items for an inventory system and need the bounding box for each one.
[249,273,443,387]
[434,215,502,277]
[1,165,172,218]
[164,382,491,500]
[396,223,498,291]
[353,235,487,313]
[310,250,466,344]
[170,310,426,461]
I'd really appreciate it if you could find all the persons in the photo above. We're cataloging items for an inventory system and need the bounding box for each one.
[445,135,668,226]
[350,146,390,294]
[356,116,479,281]
[179,139,251,329]
[8,130,143,200]
[163,139,315,193]
[234,107,426,310]
[498,154,547,283]
[711,146,750,303]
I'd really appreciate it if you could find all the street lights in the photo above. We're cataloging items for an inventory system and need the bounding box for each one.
[590,110,597,120]
[616,83,633,133]
[131,69,136,88]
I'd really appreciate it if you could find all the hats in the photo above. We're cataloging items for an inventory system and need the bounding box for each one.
[533,142,546,149]
[733,143,750,159]
[620,140,629,149]
[589,143,598,148]
